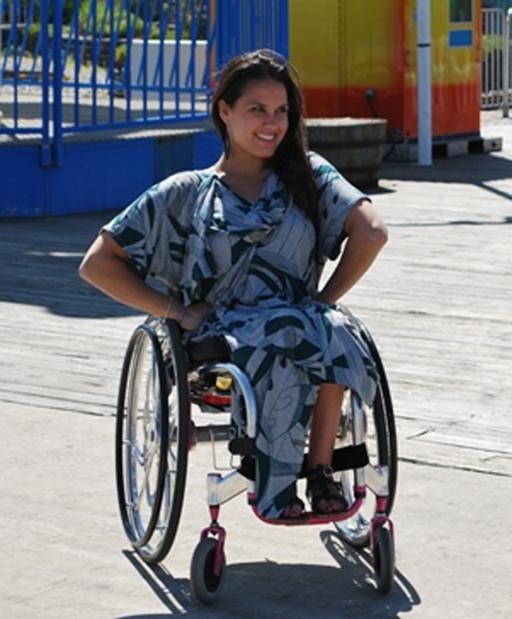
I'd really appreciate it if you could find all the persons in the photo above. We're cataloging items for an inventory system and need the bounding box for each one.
[78,49,387,518]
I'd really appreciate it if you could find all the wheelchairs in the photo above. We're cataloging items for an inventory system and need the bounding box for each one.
[115,305,398,604]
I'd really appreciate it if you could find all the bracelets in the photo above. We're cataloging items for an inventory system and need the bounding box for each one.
[176,307,186,328]
[160,296,174,325]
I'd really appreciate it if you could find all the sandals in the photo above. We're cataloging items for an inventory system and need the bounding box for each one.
[307,464,349,515]
[279,496,305,518]
